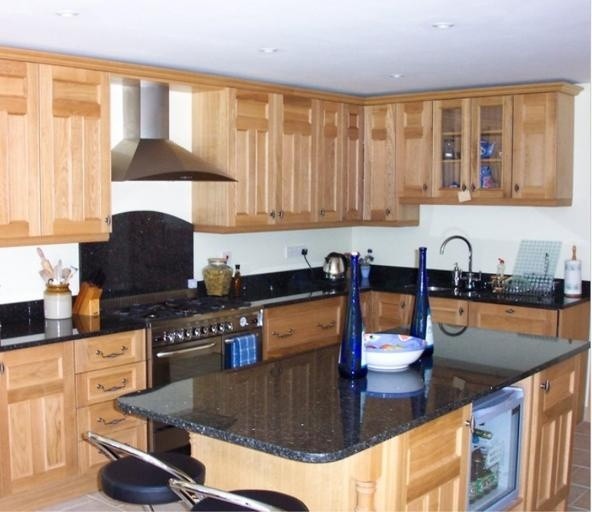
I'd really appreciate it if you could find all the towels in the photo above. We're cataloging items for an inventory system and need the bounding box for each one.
[232,334,257,369]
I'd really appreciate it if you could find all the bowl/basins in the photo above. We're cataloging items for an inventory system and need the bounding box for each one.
[361,333,428,373]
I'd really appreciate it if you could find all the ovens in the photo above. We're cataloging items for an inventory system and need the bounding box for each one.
[150,306,263,387]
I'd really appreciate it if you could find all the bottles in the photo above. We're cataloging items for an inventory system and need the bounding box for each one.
[443,138,455,159]
[233,264,242,294]
[410,361,434,419]
[469,469,498,502]
[337,251,369,380]
[497,257,504,276]
[474,428,492,439]
[411,245,436,360]
[337,377,368,449]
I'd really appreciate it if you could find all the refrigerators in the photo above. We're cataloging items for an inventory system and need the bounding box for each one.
[465,386,525,512]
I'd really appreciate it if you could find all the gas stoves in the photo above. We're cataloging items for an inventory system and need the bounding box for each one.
[100,295,250,324]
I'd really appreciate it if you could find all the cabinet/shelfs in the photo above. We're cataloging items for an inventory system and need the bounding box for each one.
[1,340,75,511]
[426,296,468,327]
[75,329,150,498]
[357,290,415,334]
[384,401,474,512]
[519,349,580,512]
[0,45,113,247]
[468,300,591,424]
[263,296,347,361]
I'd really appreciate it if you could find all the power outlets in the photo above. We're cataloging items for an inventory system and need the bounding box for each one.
[284,243,308,260]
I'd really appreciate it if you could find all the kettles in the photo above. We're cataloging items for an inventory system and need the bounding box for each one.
[323,251,347,284]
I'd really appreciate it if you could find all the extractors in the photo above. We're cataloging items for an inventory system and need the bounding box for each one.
[109,74,239,182]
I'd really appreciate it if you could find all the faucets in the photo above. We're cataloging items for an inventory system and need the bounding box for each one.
[439,235,482,289]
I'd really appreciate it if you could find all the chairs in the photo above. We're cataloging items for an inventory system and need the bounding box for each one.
[81,431,206,511]
[167,477,310,511]
